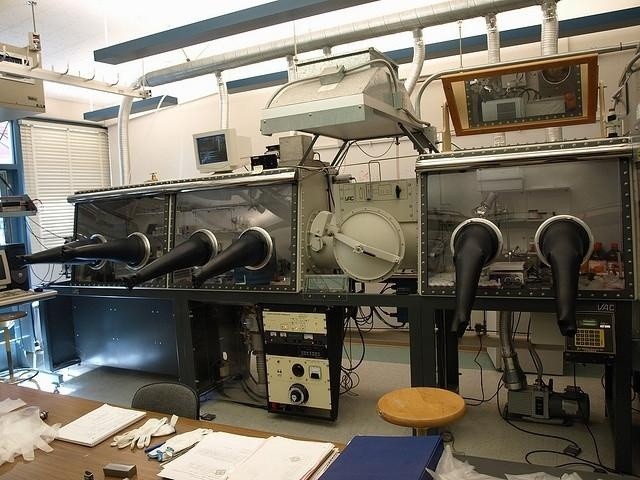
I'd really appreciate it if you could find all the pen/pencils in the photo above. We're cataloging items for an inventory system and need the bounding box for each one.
[144,441,164,453]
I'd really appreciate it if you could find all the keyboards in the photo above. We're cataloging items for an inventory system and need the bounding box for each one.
[0,289,37,301]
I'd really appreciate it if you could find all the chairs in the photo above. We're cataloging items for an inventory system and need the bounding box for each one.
[131,381,199,419]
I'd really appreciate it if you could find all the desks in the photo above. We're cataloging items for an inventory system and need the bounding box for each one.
[0,289,58,346]
[1,383,346,479]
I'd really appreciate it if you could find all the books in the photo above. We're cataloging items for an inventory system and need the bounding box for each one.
[57,401,147,450]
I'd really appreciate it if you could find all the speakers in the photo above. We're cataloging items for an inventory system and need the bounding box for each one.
[0,243,30,291]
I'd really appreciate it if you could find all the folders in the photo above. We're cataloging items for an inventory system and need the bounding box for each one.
[317,435,444,480]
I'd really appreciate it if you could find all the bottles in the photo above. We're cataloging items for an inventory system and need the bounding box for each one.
[589,242,624,275]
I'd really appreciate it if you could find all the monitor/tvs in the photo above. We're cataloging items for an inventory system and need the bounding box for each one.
[0,250,12,290]
[192,128,252,173]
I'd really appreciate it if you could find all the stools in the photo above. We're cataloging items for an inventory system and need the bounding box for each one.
[376,387,465,436]
[0,312,28,376]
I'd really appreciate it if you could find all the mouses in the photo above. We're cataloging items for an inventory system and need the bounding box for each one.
[34,288,43,292]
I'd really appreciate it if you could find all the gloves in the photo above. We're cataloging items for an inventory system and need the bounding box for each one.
[111,415,178,450]
[147,427,213,461]
